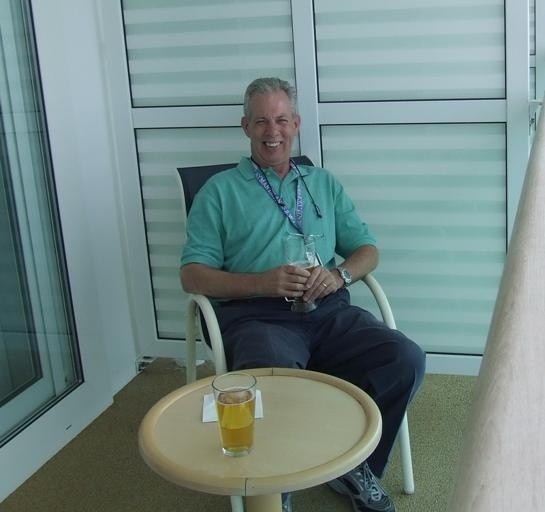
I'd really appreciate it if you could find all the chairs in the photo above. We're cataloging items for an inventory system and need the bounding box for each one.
[176,155,416,512]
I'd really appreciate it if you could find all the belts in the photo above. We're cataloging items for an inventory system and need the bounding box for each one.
[217,298,293,306]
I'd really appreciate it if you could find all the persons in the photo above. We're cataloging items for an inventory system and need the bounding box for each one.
[180,79,425,512]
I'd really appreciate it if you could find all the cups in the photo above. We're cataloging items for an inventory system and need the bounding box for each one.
[212,372,258,457]
[281,236,318,314]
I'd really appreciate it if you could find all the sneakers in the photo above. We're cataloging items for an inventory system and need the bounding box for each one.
[327,462,394,510]
[282,493,292,512]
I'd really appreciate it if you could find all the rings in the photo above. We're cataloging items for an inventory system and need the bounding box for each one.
[320,283,327,289]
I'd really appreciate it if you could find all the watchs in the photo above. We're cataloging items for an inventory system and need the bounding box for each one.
[335,267,353,290]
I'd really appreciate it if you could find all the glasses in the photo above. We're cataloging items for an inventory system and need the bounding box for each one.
[284,204,325,244]
